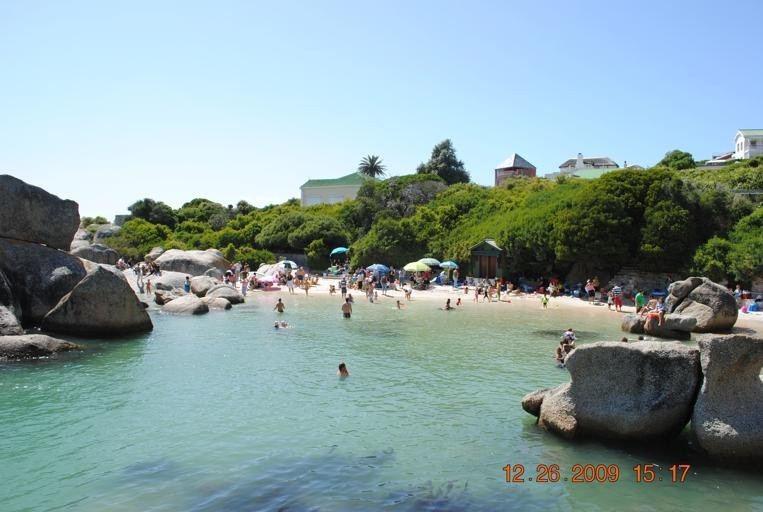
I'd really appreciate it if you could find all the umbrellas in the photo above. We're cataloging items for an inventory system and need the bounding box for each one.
[329,246,350,257]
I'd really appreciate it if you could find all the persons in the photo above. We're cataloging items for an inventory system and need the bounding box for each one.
[556,328,578,368]
[728,284,760,314]
[183,275,192,293]
[337,362,349,377]
[445,269,512,308]
[514,273,623,311]
[276,298,285,312]
[225,261,319,296]
[622,286,667,344]
[115,255,162,294]
[326,258,430,318]
[275,320,287,327]
